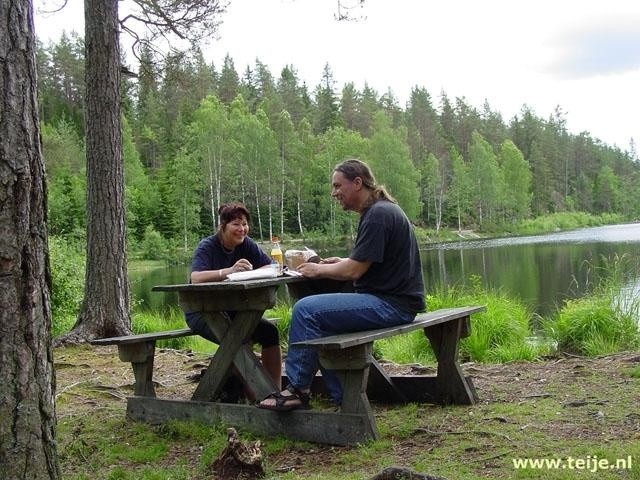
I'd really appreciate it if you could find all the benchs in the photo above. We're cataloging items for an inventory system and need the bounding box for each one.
[289,306,489,442]
[92,316,283,398]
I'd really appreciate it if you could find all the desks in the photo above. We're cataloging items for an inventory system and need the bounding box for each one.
[152,266,330,401]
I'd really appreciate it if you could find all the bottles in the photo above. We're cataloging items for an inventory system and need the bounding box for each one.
[270,236,283,277]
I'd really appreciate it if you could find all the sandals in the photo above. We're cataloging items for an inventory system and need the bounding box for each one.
[255,383,313,412]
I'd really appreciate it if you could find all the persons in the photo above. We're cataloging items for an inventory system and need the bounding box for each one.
[184,159,427,411]
